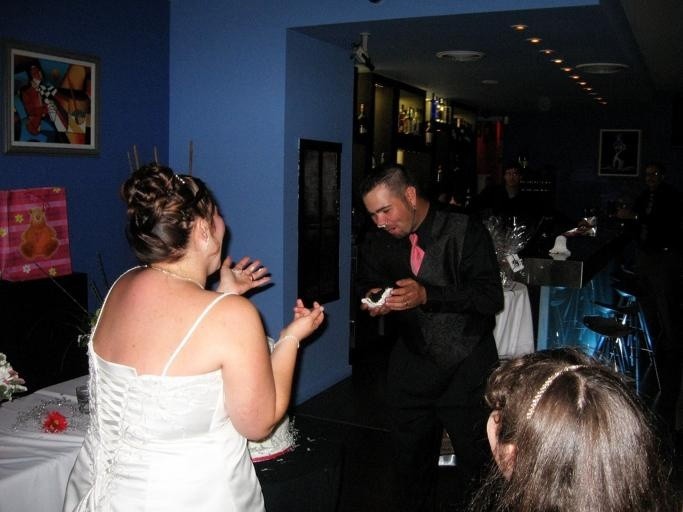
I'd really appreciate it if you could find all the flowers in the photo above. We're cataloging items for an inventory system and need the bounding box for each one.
[0,352,28,402]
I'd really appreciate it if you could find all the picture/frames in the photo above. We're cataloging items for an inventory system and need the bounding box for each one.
[0,39,101,159]
[597,128,641,178]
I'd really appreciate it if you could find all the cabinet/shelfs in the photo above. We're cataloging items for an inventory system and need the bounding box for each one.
[352,73,478,208]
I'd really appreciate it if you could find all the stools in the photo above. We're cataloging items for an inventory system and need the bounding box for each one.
[581,266,663,403]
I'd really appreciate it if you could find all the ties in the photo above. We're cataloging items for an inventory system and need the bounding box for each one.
[409,233,424,276]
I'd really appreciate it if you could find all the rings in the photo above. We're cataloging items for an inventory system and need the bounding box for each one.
[402,301,410,309]
[248,273,256,281]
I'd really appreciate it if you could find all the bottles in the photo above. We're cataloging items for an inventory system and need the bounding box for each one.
[430,92,454,124]
[358,103,367,135]
[399,105,418,134]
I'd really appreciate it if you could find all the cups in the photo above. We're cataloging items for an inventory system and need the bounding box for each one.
[76,385,89,415]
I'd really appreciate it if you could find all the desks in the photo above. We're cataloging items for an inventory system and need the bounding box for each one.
[0,373,91,512]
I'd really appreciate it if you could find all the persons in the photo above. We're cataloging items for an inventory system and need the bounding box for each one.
[477,160,539,221]
[454,344,682,512]
[358,163,507,512]
[63,160,325,511]
[608,162,679,281]
[22,65,61,140]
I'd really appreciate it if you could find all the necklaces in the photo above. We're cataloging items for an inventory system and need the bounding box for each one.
[148,267,205,290]
